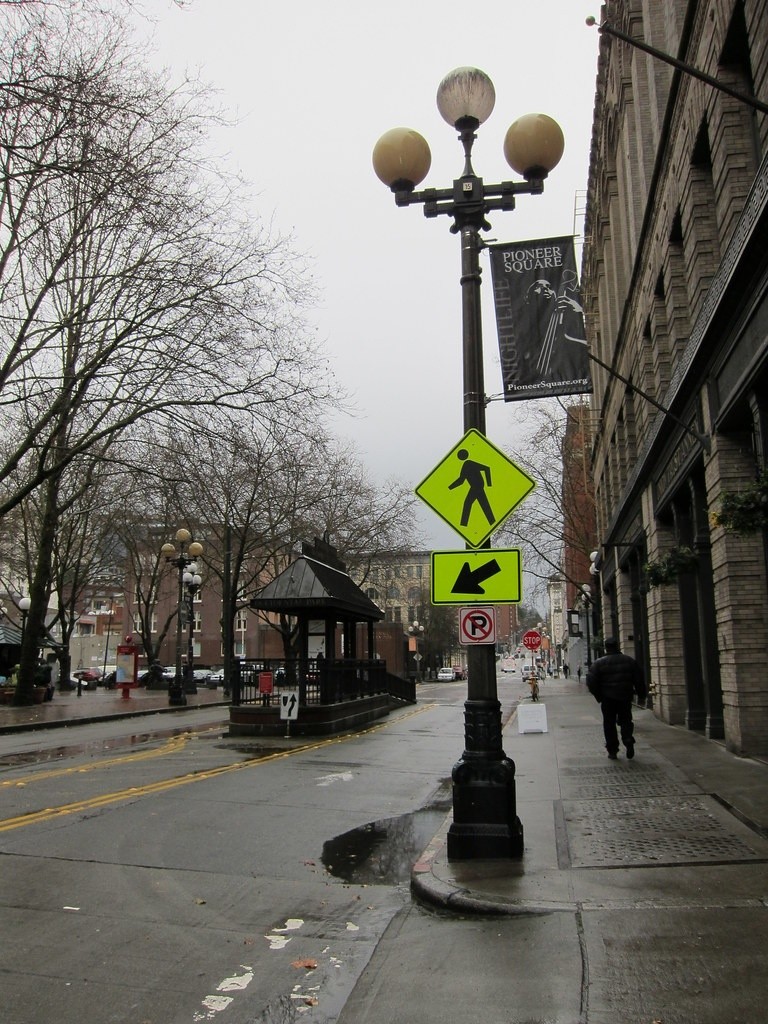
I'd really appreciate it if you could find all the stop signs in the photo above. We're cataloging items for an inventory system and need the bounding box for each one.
[522,631,543,650]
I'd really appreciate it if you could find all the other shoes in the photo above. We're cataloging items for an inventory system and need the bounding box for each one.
[625,740,634,759]
[608,753,617,759]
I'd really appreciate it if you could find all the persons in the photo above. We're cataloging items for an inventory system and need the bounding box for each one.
[586,637,646,760]
[563,664,568,679]
[317,652,324,669]
[38,666,55,702]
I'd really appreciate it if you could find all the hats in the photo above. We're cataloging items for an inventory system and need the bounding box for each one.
[606,638,618,649]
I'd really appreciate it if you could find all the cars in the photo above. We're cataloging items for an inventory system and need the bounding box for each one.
[72,659,296,689]
[436,667,456,683]
[501,641,544,682]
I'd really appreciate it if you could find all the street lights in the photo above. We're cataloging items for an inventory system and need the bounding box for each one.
[182,563,202,694]
[160,528,203,707]
[19,596,31,643]
[579,584,592,685]
[370,64,565,863]
